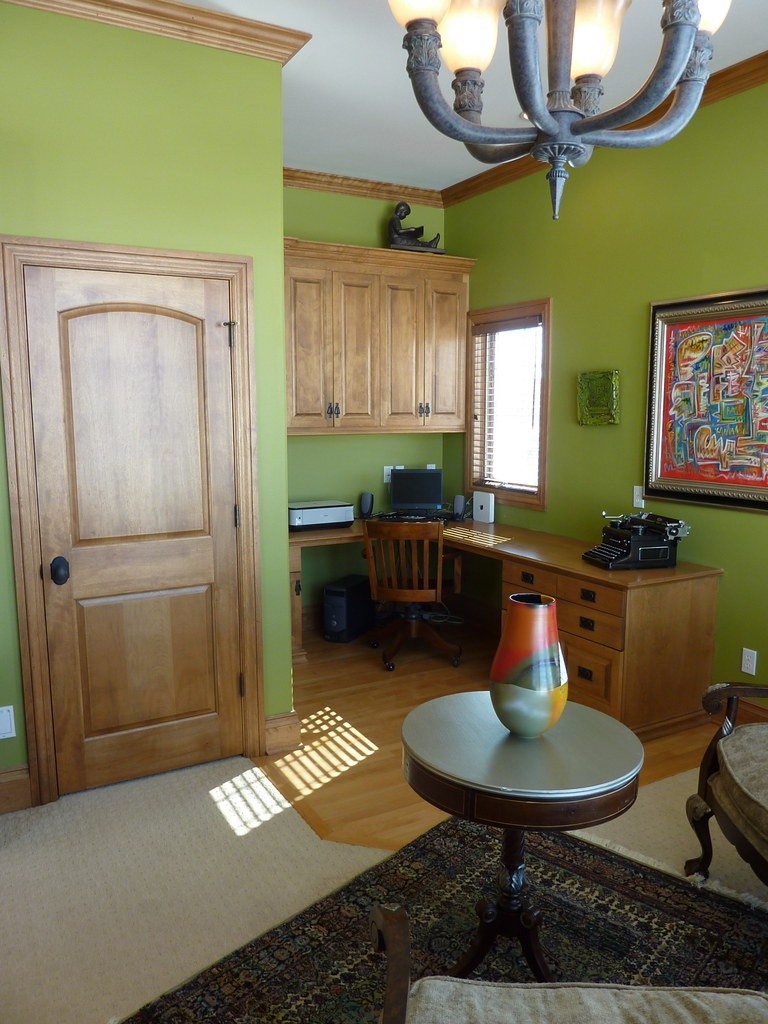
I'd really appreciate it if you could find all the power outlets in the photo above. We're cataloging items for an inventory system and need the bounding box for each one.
[0,704,16,739]
[633,486,645,508]
[427,464,436,469]
[384,466,404,484]
[741,648,757,676]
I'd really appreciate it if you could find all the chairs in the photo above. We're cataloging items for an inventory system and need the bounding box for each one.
[685,681,768,891]
[363,519,461,670]
[363,900,768,1024]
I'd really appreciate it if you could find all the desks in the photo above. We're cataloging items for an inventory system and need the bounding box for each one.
[402,690,645,982]
[289,517,585,664]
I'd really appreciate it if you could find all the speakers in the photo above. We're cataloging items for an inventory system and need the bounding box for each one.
[452,495,466,522]
[359,492,374,519]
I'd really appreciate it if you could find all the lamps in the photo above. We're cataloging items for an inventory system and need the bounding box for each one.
[386,0,731,220]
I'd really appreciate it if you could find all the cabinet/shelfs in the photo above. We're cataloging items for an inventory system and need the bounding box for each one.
[501,560,723,743]
[282,236,476,435]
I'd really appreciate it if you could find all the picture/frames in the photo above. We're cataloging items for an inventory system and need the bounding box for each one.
[643,287,768,514]
[577,369,620,425]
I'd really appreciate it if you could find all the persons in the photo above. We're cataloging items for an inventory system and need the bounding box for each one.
[387,201,446,254]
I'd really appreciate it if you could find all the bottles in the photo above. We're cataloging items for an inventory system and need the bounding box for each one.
[488,592,569,741]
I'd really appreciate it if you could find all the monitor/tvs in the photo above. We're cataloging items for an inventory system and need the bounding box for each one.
[390,469,443,509]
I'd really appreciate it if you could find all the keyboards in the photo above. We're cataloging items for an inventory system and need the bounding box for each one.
[379,514,450,525]
[584,542,627,562]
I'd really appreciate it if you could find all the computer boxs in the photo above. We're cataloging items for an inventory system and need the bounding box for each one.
[322,574,368,642]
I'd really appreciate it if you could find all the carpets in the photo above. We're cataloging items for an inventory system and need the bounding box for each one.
[116,814,768,1024]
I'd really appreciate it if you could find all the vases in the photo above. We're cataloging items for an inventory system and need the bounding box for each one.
[490,592,569,739]
